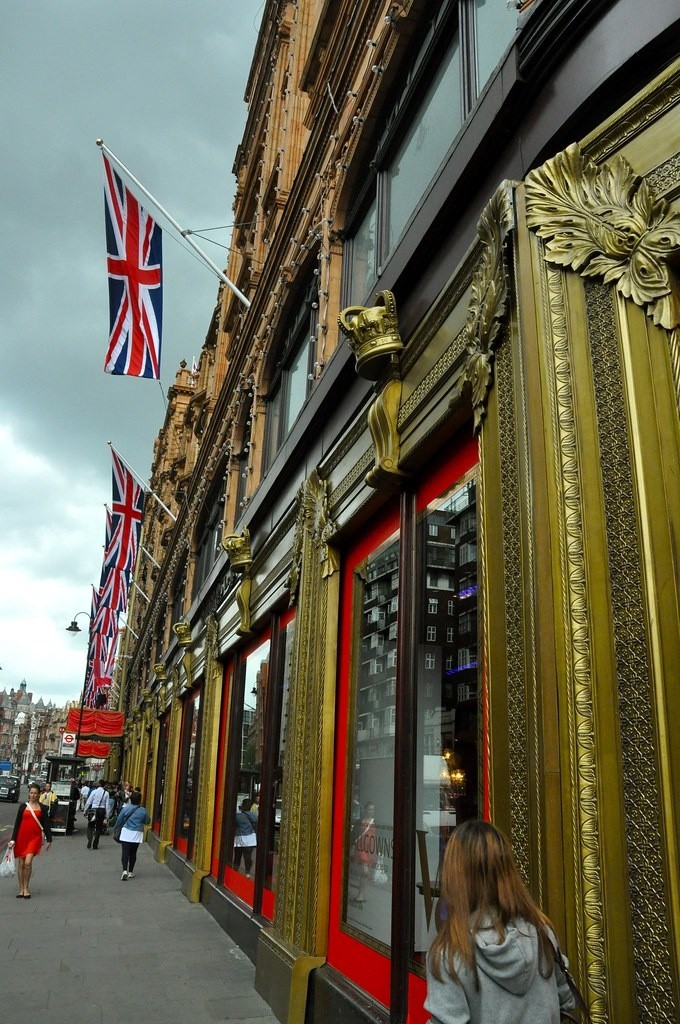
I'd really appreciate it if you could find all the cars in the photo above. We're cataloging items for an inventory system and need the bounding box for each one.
[27,771,48,791]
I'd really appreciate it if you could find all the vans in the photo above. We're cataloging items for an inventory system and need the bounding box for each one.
[0,776,21,803]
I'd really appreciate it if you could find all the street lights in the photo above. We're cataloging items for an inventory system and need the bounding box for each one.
[63,613,95,837]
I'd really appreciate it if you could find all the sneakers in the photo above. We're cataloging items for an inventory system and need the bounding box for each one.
[128,872,135,878]
[121,871,128,881]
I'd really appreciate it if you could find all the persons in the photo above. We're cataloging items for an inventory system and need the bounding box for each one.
[350,801,378,903]
[423,819,582,1024]
[233,792,260,878]
[76,780,151,881]
[8,782,59,899]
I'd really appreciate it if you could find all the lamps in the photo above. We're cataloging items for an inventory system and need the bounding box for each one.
[66,612,91,638]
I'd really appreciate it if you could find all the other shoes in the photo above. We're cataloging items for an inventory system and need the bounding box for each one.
[92,846,98,849]
[24,894,31,899]
[87,839,91,848]
[16,894,23,898]
[245,874,251,879]
[355,898,367,903]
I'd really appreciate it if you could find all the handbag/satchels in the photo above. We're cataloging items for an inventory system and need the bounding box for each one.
[373,855,387,887]
[113,826,122,844]
[348,845,356,860]
[0,841,16,879]
[86,809,98,822]
[41,831,45,846]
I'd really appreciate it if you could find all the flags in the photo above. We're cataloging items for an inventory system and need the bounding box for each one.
[81,448,146,711]
[101,155,163,380]
